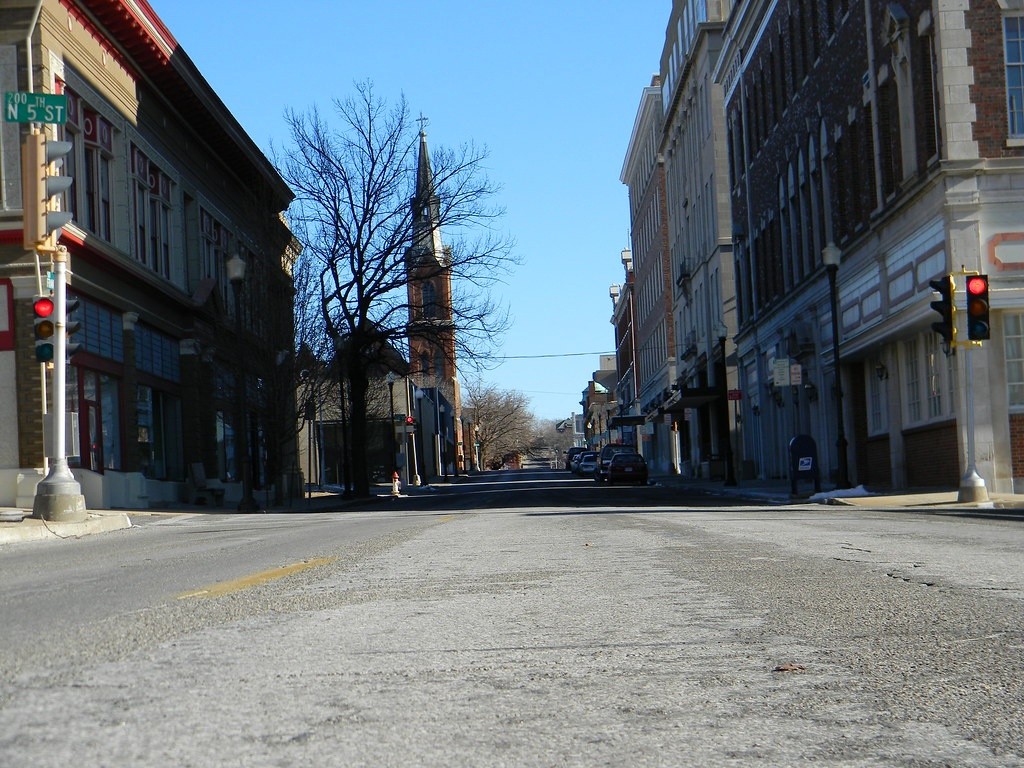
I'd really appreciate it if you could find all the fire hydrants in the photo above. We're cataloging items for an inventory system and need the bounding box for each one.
[390,471,400,494]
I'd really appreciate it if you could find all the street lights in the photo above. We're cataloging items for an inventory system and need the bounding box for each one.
[605,402,613,444]
[618,397,626,447]
[440,404,450,482]
[388,371,401,491]
[416,388,429,486]
[467,419,474,471]
[592,416,596,435]
[597,410,603,450]
[475,424,481,468]
[822,238,853,490]
[716,321,738,488]
[459,414,468,474]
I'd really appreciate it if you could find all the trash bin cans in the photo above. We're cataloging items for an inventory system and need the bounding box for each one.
[709,454,724,481]
[789,434,821,496]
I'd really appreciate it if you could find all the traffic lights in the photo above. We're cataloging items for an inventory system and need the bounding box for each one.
[34,293,56,365]
[406,416,415,437]
[927,275,953,345]
[27,134,73,244]
[64,299,84,364]
[963,274,990,341]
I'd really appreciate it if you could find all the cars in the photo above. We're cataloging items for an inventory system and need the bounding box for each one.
[569,454,579,469]
[573,451,600,475]
[577,454,600,477]
[607,453,649,485]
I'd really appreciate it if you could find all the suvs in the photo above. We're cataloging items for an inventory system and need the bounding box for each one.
[596,443,638,483]
[563,447,587,471]
